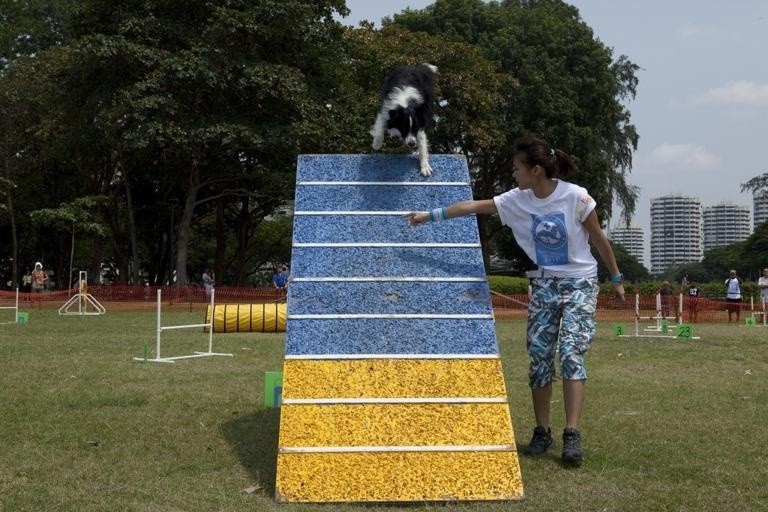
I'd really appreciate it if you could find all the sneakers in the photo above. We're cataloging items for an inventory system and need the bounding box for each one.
[561,427,582,464]
[526,425,552,454]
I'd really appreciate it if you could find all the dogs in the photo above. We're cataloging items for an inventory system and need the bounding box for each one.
[369,61,448,179]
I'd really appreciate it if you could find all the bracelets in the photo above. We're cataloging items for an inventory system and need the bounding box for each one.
[609,273,625,284]
[429,207,447,222]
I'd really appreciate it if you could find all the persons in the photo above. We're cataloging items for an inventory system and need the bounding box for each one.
[272,264,287,300]
[201,267,216,301]
[756,267,767,325]
[685,282,702,323]
[723,269,743,323]
[21,270,32,302]
[656,280,673,318]
[29,262,49,308]
[399,136,628,471]
